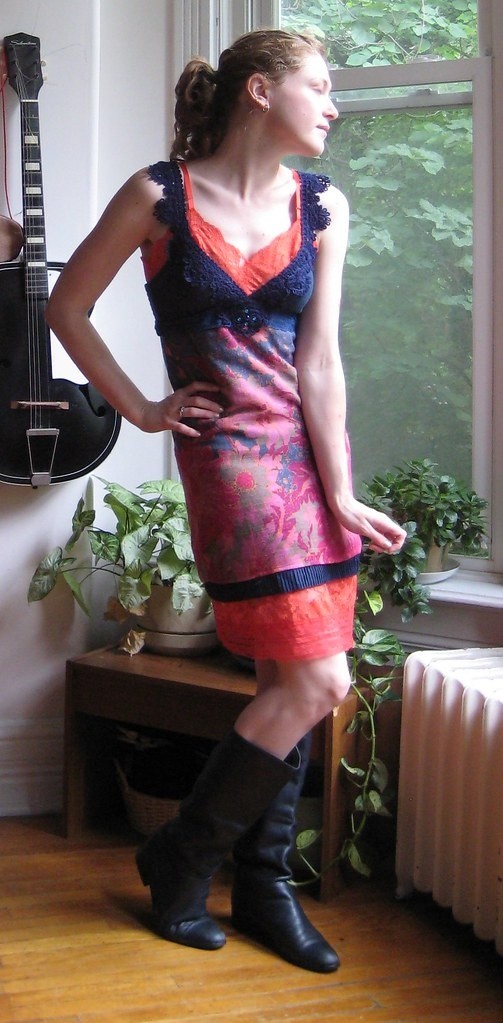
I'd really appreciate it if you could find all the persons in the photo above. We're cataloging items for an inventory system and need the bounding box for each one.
[44,29,407,973]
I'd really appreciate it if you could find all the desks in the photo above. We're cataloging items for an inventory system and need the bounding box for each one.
[63,638,412,905]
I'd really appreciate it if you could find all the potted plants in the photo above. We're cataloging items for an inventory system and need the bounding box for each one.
[353,459,494,574]
[28,478,218,659]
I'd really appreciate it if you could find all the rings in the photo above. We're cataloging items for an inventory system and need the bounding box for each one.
[179,406,184,419]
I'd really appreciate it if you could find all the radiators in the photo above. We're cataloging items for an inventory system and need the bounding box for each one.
[393,648,503,958]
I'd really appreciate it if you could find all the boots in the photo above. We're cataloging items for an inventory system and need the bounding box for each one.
[134,727,301,950]
[230,728,341,973]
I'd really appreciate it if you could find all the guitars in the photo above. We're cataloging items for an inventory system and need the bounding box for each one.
[0,30,123,489]
